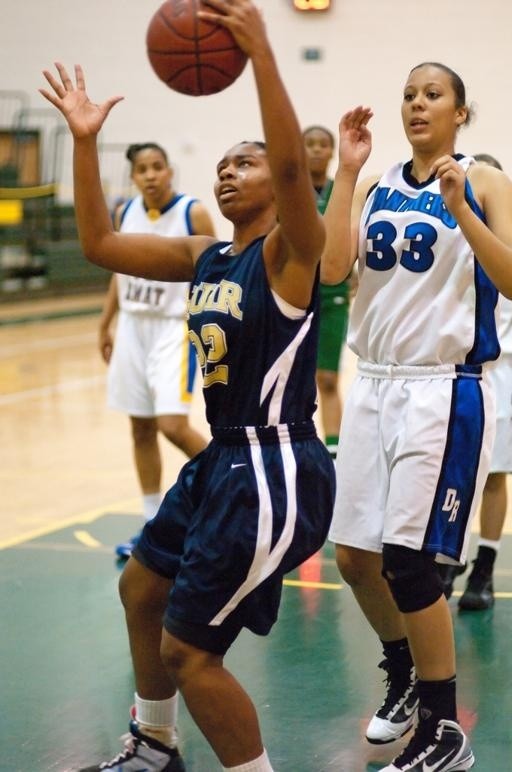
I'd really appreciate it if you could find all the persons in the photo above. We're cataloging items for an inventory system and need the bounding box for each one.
[32,0,343,772]
[456,151,508,616]
[93,140,221,562]
[271,122,361,464]
[316,58,510,772]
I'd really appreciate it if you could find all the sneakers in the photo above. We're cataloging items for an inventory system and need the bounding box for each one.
[75,719,187,772]
[363,658,420,745]
[456,557,494,609]
[373,705,477,770]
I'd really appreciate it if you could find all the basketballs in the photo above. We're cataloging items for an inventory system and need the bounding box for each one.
[145,0,248,97]
